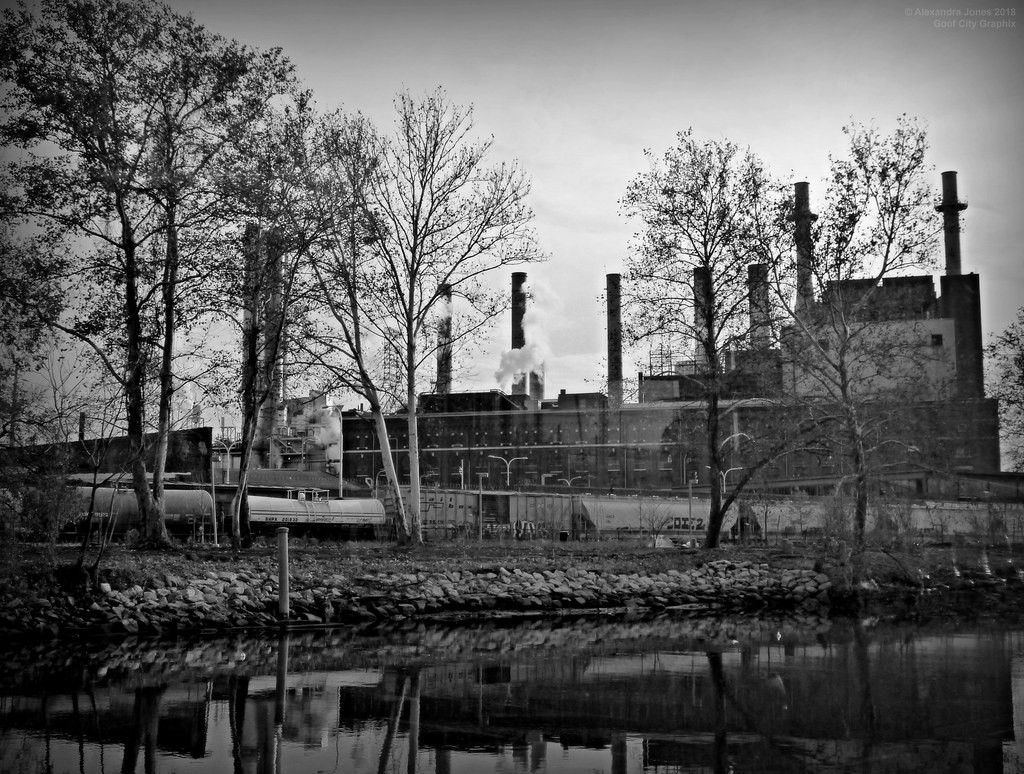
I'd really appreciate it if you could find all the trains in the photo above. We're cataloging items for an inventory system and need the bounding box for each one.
[43,486,387,550]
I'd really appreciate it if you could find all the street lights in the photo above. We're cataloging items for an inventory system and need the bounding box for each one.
[488,454,529,486]
[557,475,582,488]
[706,465,744,495]
